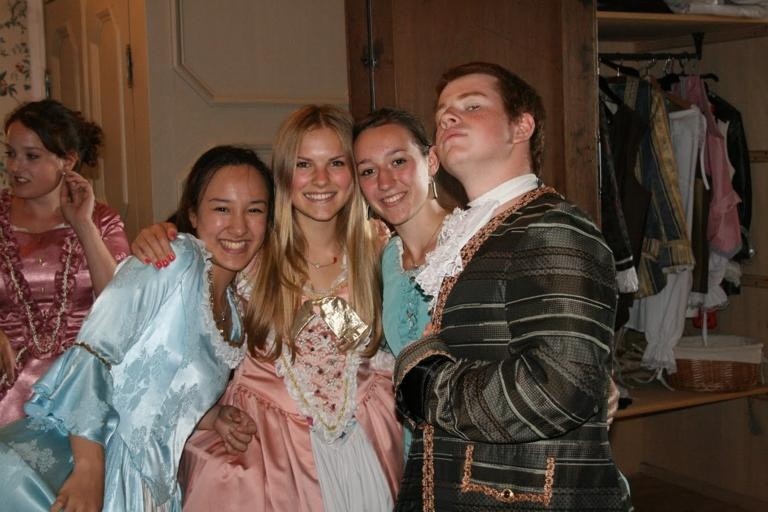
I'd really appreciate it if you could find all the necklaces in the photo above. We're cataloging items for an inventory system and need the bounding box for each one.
[212,295,231,322]
[402,218,444,267]
[300,248,342,269]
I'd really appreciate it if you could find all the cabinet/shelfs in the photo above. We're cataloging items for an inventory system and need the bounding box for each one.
[561,0,768,402]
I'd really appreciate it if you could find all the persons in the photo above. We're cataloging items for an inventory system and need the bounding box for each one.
[0,144,272,510]
[131,104,405,512]
[394,61,635,511]
[354,109,467,470]
[0,100,131,427]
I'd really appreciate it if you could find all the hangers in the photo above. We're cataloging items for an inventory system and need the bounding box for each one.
[597,50,720,109]
